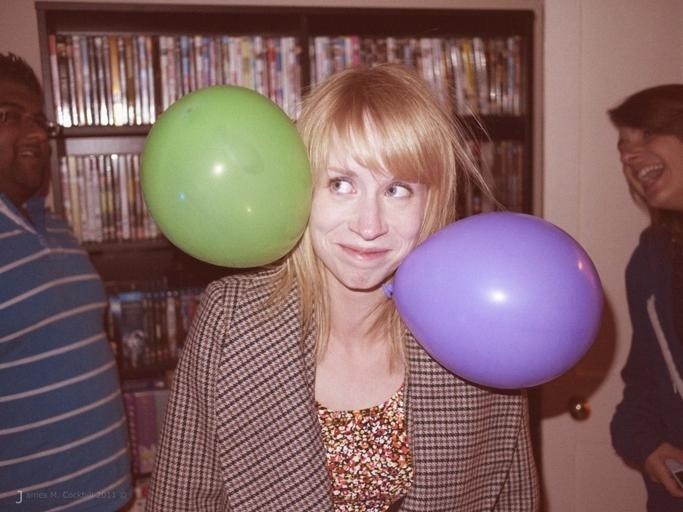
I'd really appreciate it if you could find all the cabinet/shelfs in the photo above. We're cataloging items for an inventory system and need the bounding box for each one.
[38,0,544,511]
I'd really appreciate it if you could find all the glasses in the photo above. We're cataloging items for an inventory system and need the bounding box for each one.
[0,107,60,139]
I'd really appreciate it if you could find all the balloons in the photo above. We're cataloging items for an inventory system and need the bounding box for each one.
[139,85,312,270]
[381,212,606,391]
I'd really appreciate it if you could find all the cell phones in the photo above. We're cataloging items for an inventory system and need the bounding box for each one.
[665,458,683,490]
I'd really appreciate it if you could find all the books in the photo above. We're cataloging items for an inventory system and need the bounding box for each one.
[61,154,157,238]
[47,30,530,128]
[108,286,199,512]
[456,140,528,212]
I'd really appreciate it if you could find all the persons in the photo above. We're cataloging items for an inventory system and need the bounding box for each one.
[1,48,135,512]
[146,60,544,512]
[606,83,682,511]
